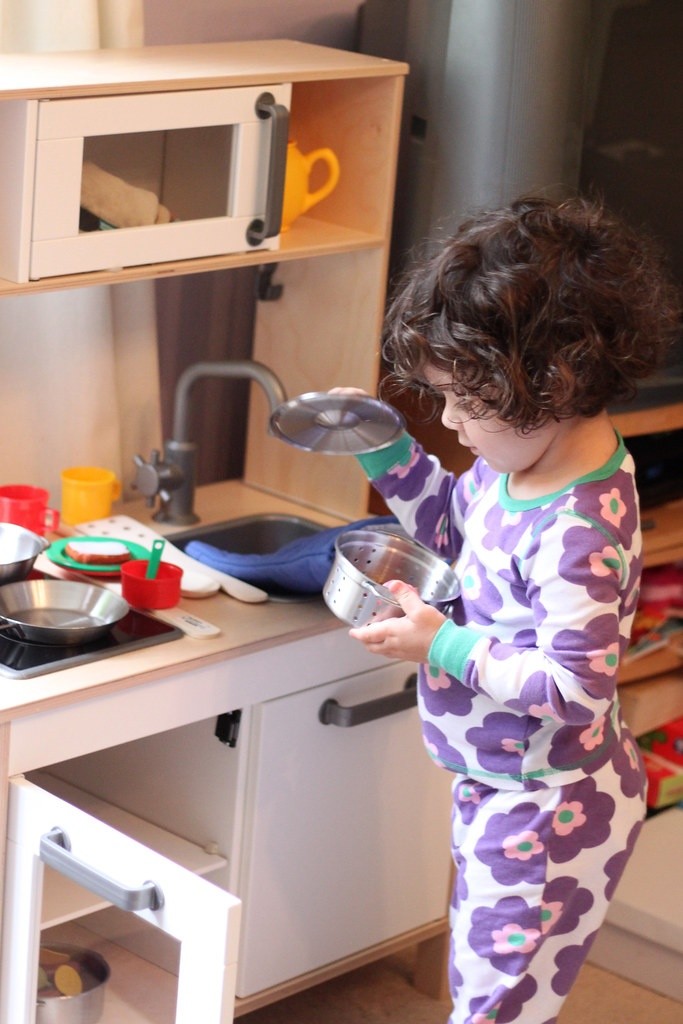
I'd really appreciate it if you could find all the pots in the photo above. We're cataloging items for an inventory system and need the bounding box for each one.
[1,523,52,580]
[0,580,130,647]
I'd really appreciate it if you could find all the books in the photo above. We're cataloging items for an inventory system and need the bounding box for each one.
[633,723,683,810]
[619,620,683,665]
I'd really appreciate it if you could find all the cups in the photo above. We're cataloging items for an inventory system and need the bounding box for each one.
[1,485,60,537]
[61,469,122,525]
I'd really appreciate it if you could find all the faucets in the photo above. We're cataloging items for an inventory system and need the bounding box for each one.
[128,360,289,527]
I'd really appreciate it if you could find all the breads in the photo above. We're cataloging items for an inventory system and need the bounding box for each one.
[65,541,132,564]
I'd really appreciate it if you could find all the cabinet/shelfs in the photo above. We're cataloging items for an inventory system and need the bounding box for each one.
[0,628,459,1024]
[0,36,410,299]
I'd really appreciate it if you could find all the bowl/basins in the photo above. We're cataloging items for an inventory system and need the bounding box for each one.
[35,942,112,1024]
[121,559,183,611]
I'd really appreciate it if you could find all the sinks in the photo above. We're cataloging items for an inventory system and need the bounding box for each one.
[158,513,354,605]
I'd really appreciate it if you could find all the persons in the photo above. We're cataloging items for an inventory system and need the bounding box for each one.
[328,193,669,1024]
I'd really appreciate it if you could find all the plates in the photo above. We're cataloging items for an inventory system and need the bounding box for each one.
[48,535,150,578]
[178,571,219,599]
